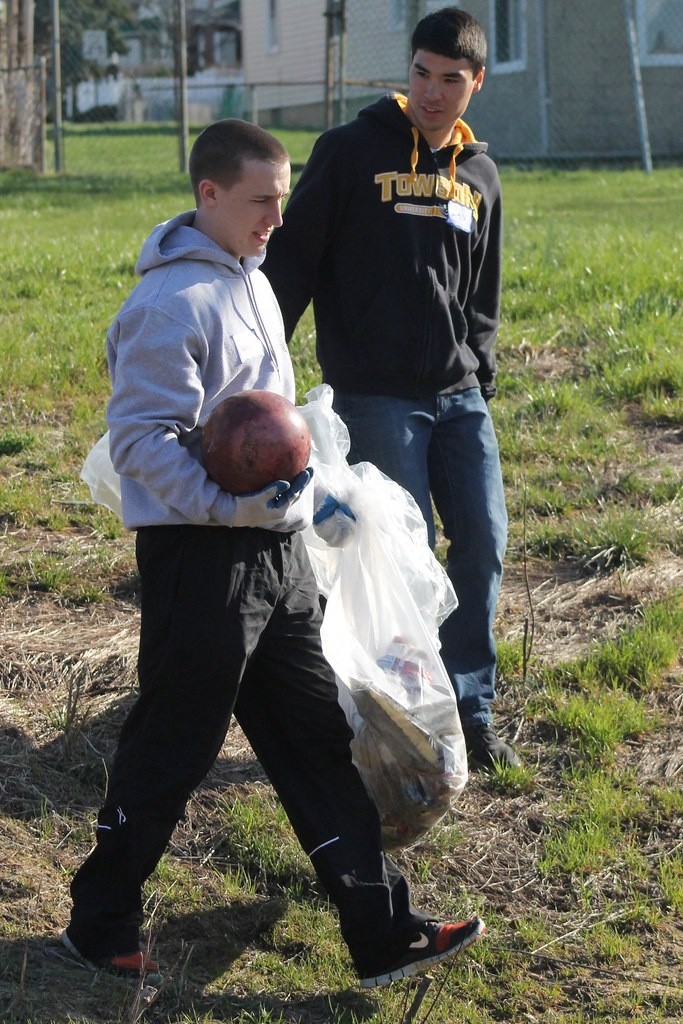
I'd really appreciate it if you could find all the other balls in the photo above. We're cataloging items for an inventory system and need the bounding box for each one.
[201,390,309,495]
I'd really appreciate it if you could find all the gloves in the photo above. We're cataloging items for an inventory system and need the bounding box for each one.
[232,466,314,526]
[314,485,356,527]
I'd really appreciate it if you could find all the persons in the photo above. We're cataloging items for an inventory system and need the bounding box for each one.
[62,118,486,990]
[256,6,531,786]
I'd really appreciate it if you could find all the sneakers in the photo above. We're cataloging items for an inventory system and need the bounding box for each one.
[464,724,521,771]
[355,915,488,988]
[60,926,164,986]
